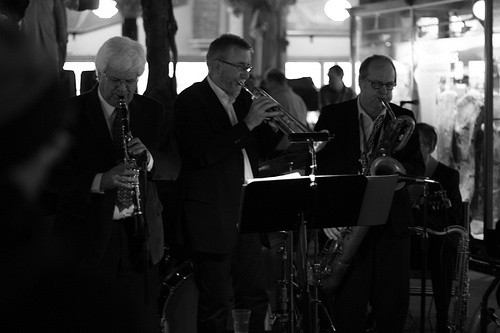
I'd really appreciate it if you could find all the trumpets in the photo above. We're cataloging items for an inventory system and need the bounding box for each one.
[238,79,334,154]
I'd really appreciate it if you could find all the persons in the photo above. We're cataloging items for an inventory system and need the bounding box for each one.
[265,69,308,159]
[65,36,173,272]
[412,122,463,333]
[452,73,483,206]
[1,71,144,333]
[169,34,290,333]
[319,65,357,110]
[305,55,425,333]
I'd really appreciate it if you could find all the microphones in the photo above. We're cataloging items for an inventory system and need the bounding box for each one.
[402,99,419,104]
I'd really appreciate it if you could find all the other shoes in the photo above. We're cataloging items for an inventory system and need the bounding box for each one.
[436,319,456,332]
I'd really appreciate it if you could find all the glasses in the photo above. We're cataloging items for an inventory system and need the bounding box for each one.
[366,79,397,90]
[222,60,254,73]
[104,72,138,85]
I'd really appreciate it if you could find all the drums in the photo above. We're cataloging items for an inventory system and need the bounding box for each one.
[159,265,199,333]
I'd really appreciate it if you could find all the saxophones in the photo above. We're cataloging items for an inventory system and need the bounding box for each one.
[307,97,417,292]
[118,94,144,233]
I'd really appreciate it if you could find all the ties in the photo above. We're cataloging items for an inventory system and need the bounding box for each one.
[112,108,136,208]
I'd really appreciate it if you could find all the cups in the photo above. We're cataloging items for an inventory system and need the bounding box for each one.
[232,309,251,333]
